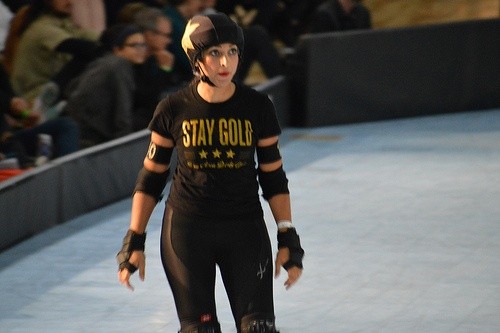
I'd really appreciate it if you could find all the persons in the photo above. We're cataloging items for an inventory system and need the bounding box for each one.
[0,0,371,166]
[116,15,304,333]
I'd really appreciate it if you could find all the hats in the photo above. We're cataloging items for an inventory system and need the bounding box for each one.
[181,13,244,74]
[108,22,141,49]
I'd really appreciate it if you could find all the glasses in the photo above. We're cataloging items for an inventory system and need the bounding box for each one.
[123,41,147,49]
[151,28,176,39]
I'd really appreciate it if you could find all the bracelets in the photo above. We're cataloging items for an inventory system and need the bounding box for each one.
[278,220,292,229]
